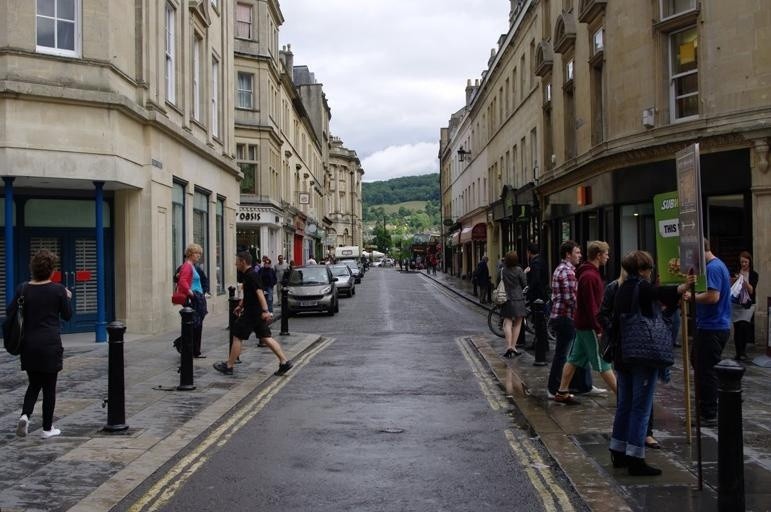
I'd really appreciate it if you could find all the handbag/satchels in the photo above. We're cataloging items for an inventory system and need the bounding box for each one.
[491,280,509,305]
[596,331,615,363]
[171,292,187,305]
[619,313,676,366]
[730,273,752,310]
[3,281,27,357]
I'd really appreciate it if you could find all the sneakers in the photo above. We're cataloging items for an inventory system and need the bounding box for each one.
[213,361,234,375]
[274,360,294,376]
[196,354,206,358]
[16,415,29,438]
[611,448,662,476]
[544,382,607,406]
[41,427,62,439]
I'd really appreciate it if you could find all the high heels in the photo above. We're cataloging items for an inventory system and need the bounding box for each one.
[504,347,522,359]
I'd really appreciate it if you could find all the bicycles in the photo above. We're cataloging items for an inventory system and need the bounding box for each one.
[488,278,557,341]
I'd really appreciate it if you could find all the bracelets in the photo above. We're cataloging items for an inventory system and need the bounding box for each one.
[261,309,269,313]
[238,304,242,310]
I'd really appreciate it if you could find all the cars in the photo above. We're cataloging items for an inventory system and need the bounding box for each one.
[283,247,396,316]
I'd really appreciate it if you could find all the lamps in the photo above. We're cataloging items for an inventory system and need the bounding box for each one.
[456,145,471,162]
[640,105,661,129]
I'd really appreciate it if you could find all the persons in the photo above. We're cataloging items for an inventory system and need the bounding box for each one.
[172,240,209,362]
[254,244,372,318]
[12,247,76,440]
[383,253,439,277]
[172,252,210,298]
[474,254,494,306]
[212,250,294,377]
